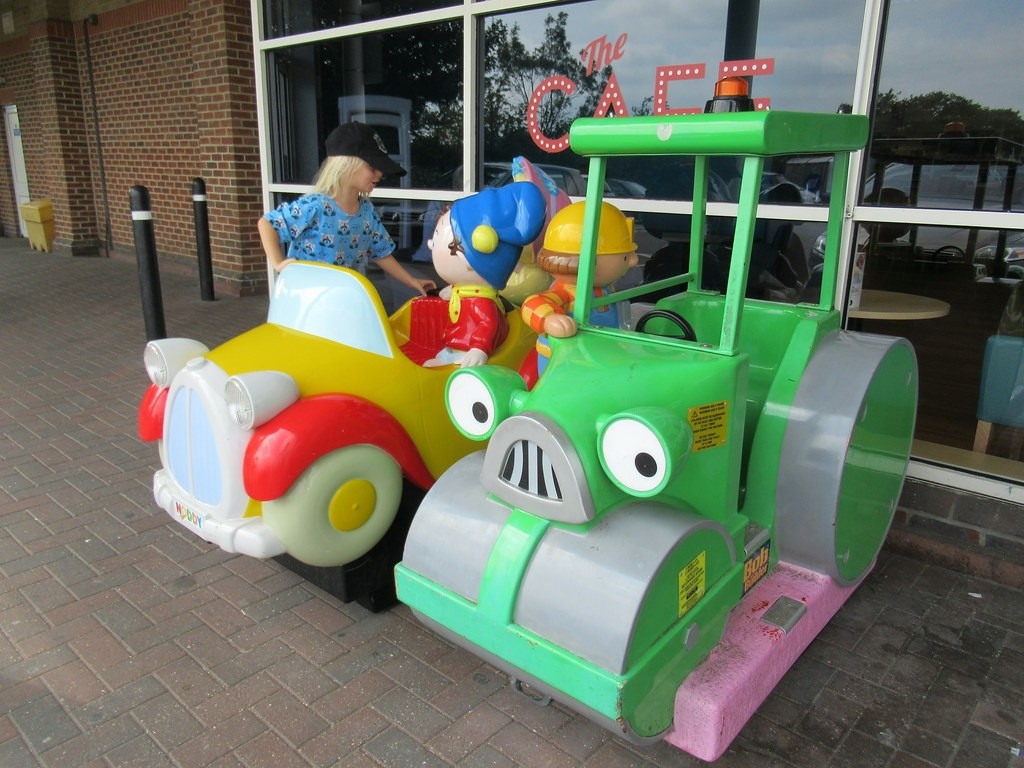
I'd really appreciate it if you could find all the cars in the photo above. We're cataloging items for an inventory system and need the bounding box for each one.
[479,161,649,226]
[390,115,917,768]
[724,156,1024,291]
[141,276,589,617]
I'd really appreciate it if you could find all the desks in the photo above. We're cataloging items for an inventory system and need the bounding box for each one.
[848,290,950,332]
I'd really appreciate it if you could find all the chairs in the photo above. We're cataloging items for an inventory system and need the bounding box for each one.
[972,335,1024,453]
[397,297,448,366]
[647,290,840,526]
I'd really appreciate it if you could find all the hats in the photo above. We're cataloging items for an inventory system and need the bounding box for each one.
[326,120,408,178]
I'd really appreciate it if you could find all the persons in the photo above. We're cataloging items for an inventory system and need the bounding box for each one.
[422,181,548,370]
[257,120,438,298]
[522,197,639,379]
